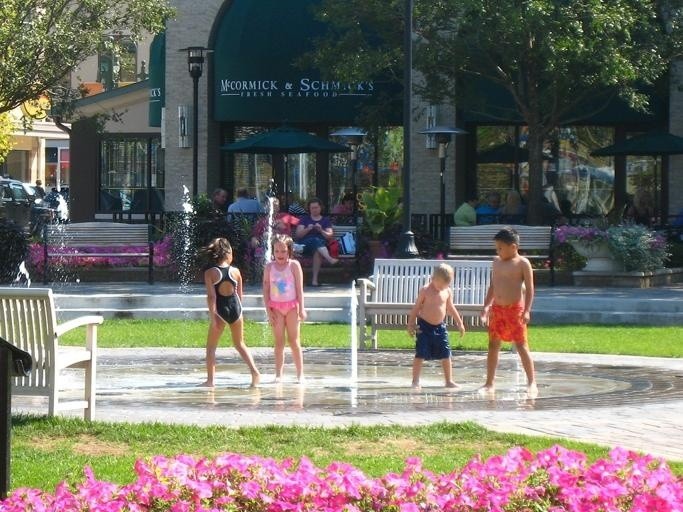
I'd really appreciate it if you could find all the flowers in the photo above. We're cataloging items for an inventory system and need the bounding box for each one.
[552,217,674,273]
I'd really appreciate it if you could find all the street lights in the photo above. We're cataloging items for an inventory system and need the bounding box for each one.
[177,46,214,203]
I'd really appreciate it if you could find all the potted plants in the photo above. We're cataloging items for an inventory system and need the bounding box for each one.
[355,171,405,259]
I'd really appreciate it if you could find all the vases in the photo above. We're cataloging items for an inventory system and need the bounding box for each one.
[567,239,626,273]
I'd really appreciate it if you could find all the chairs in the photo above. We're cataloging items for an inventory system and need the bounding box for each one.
[1,286,108,425]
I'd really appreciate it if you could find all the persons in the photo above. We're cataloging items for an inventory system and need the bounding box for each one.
[251,198,339,286]
[477,228,540,398]
[453,191,527,225]
[35,179,46,199]
[197,237,261,387]
[263,234,307,384]
[333,193,354,214]
[210,189,227,209]
[280,193,306,214]
[408,263,465,389]
[227,187,263,223]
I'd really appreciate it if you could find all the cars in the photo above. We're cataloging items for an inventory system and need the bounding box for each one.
[0,178,55,221]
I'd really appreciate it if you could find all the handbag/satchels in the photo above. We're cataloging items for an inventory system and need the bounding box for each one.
[327,231,356,258]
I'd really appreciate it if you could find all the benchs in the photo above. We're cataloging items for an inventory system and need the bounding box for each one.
[40,221,157,287]
[358,254,494,352]
[442,223,557,287]
[293,225,359,272]
